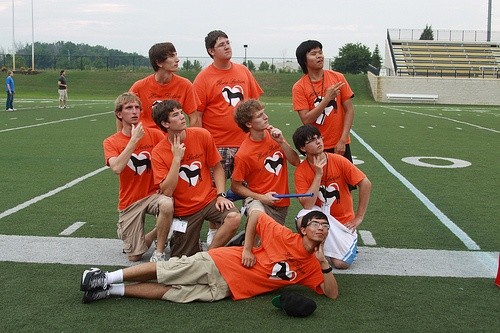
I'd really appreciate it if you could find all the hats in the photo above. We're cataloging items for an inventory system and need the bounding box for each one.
[272,290,317,318]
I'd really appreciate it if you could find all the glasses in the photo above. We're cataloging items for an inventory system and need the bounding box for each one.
[213,41,231,49]
[306,223,330,230]
[305,136,322,144]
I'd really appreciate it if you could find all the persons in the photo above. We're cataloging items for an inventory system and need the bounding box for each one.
[5,71,16,112]
[291,40,357,201]
[115,43,202,133]
[192,30,264,247]
[57,69,70,109]
[225,98,301,248]
[102,92,175,263]
[151,99,242,259]
[79,211,339,305]
[292,125,372,269]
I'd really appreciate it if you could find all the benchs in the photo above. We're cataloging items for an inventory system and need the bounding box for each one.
[402,40,500,79]
[386,93,439,105]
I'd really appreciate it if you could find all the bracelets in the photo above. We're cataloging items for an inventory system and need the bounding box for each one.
[322,265,332,273]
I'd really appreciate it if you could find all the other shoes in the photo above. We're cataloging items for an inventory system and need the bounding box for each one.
[59,105,66,109]
[64,105,69,109]
[7,108,16,111]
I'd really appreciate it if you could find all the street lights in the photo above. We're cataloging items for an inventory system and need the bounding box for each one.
[244,44,248,67]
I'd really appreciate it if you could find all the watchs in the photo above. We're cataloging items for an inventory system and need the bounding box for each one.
[217,192,228,199]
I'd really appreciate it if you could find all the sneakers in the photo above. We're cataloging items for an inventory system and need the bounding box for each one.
[80,268,107,291]
[207,231,216,247]
[150,250,166,262]
[83,285,113,304]
[225,230,245,246]
